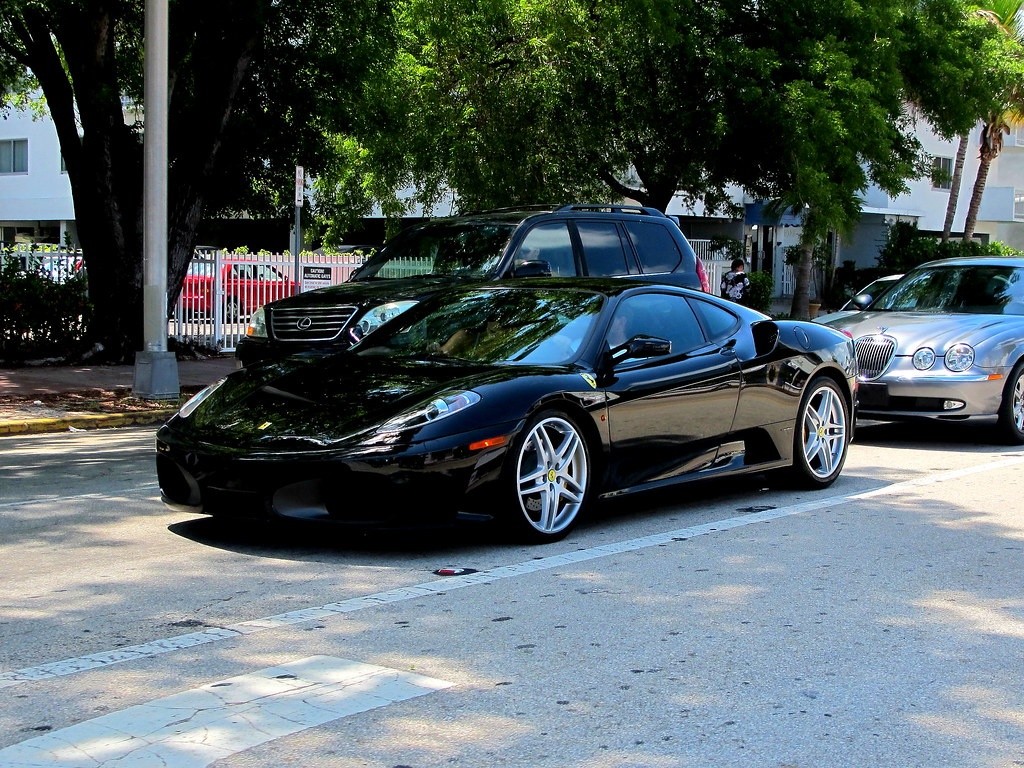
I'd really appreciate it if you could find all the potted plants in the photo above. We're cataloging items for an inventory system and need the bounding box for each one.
[808,298,822,318]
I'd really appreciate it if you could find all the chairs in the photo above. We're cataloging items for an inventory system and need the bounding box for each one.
[630,308,666,338]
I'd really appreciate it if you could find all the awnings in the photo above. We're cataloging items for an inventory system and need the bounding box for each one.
[742,194,918,225]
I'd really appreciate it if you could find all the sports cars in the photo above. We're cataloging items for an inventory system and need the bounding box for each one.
[152,274,862,544]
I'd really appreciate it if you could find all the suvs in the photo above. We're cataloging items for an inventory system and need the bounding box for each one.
[230,202,713,365]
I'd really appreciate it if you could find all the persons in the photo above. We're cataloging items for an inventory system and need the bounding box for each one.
[610,302,652,359]
[427,314,504,354]
[720,259,750,305]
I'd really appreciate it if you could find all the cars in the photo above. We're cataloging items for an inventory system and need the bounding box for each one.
[820,255,1024,442]
[175,258,302,322]
[809,274,905,326]
[14,255,82,284]
